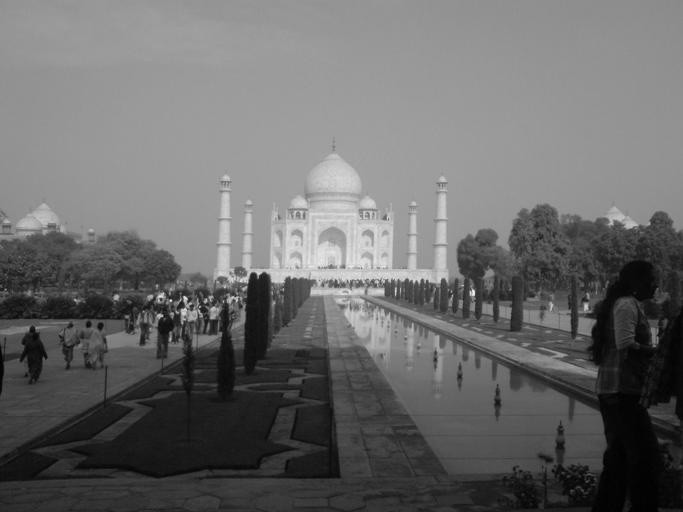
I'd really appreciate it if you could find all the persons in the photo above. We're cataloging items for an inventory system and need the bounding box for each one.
[549,293,553,312]
[470,288,475,303]
[321,278,385,288]
[583,291,590,311]
[586,259,661,512]
[638,304,683,425]
[657,316,670,342]
[18,281,284,386]
